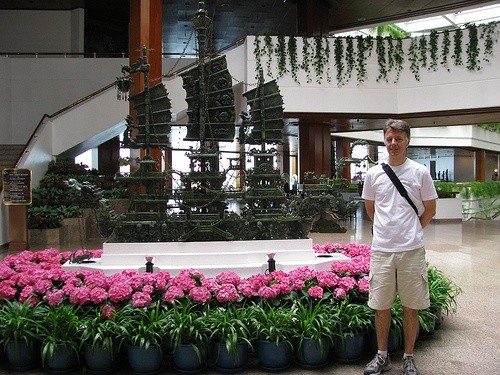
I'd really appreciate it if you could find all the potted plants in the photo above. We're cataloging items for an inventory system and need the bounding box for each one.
[23,160,132,247]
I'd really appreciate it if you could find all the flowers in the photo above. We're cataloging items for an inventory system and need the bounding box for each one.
[0,243,464,365]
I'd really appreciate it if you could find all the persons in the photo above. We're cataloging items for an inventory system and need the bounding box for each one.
[361,119,439,375]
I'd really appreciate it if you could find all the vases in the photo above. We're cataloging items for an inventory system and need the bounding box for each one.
[5,306,442,371]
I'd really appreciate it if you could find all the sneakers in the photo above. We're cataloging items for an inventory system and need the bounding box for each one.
[401,356,420,375]
[364,351,392,375]
